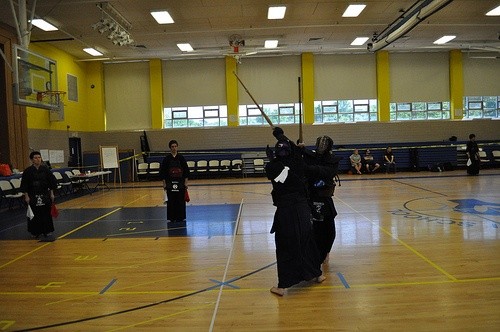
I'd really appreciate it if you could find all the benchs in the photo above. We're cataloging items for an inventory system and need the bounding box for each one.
[349,153,400,173]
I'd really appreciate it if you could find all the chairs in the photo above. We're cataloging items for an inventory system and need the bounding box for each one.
[491,147,500,169]
[0,170,86,215]
[137,159,243,180]
[253,158,266,180]
[477,148,491,169]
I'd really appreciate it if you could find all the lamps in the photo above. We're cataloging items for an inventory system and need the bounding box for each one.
[91,17,135,49]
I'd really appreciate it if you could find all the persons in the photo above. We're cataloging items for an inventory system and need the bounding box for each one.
[362,151,383,174]
[19,152,57,239]
[161,140,190,224]
[465,134,482,175]
[270,127,339,296]
[384,146,397,173]
[349,148,362,175]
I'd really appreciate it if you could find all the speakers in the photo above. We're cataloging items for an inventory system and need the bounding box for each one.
[69,137,82,166]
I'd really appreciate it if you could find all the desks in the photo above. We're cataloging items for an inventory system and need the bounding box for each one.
[73,170,113,196]
[243,155,268,176]
